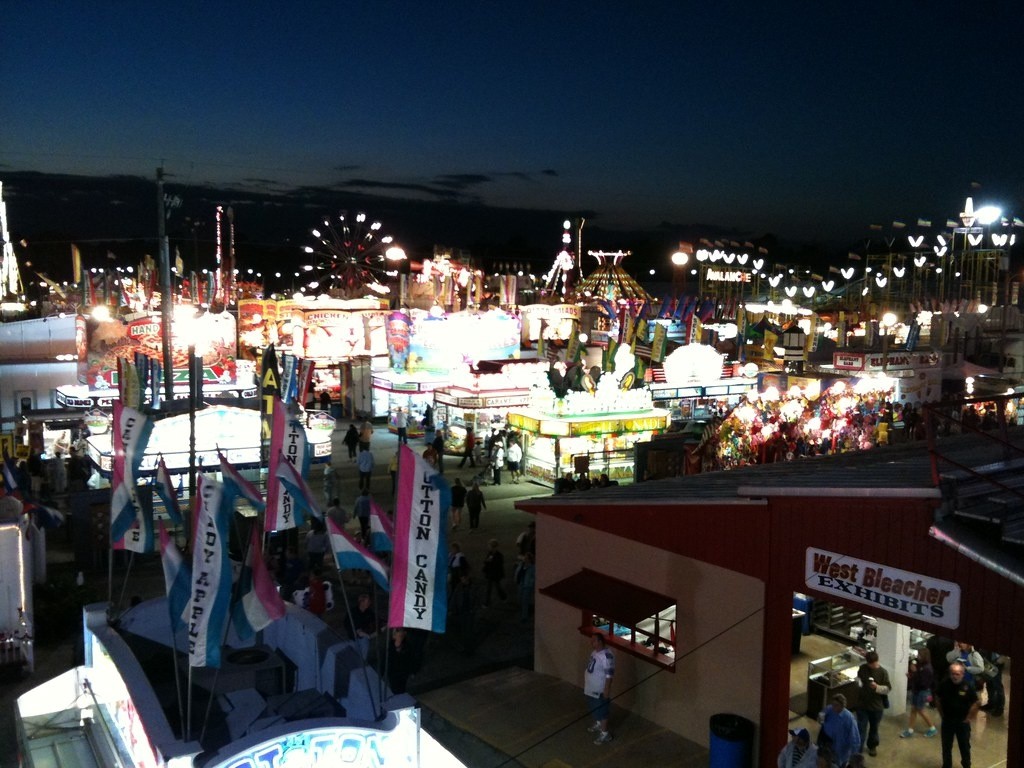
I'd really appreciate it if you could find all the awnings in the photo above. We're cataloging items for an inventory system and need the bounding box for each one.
[541,568,675,629]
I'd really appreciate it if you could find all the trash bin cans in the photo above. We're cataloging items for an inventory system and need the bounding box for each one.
[708,712,757,768]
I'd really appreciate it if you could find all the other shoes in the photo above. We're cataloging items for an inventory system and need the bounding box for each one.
[510,480,516,484]
[900,730,915,738]
[925,728,939,737]
[516,479,521,484]
[469,530,474,534]
[452,524,458,529]
[870,746,876,756]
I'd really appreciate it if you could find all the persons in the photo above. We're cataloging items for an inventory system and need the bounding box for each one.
[927,634,954,707]
[946,641,985,687]
[777,727,819,768]
[513,553,535,622]
[382,628,416,694]
[448,543,469,603]
[583,632,616,744]
[479,539,508,605]
[935,663,981,768]
[27,446,81,501]
[857,651,891,757]
[516,521,535,562]
[267,380,620,607]
[343,594,376,642]
[979,648,1007,716]
[720,396,999,471]
[816,694,862,768]
[898,659,938,737]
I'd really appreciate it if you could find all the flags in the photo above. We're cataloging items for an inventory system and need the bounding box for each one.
[805,312,922,353]
[104,342,451,667]
[538,294,776,390]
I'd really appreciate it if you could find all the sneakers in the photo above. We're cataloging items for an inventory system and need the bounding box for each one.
[587,721,601,733]
[594,732,614,745]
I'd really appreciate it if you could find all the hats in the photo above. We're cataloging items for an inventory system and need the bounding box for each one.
[830,695,848,706]
[865,651,879,664]
[494,442,503,447]
[788,727,810,741]
